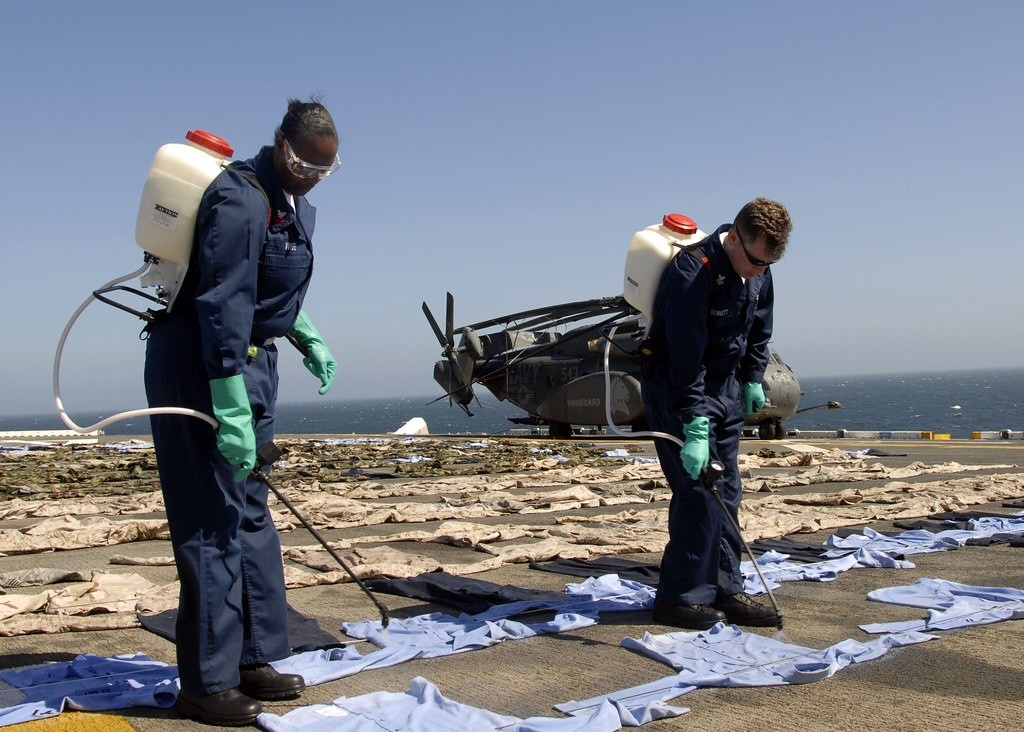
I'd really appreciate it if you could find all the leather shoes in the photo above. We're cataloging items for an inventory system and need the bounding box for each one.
[238,660,306,700]
[715,592,785,628]
[172,682,263,728]
[659,600,729,633]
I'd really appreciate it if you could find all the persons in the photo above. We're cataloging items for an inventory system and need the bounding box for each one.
[640,197,792,631]
[144,95,341,729]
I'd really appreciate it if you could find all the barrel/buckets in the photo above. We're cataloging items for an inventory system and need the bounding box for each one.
[134,130,236,270]
[622,213,709,317]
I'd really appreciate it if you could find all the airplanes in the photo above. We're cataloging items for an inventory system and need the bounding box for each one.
[421,290,843,438]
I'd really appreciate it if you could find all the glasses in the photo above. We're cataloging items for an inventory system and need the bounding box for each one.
[734,223,778,268]
[282,137,342,181]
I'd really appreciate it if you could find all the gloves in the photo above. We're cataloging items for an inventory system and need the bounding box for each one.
[680,415,711,480]
[286,305,338,396]
[205,374,256,484]
[743,377,765,416]
